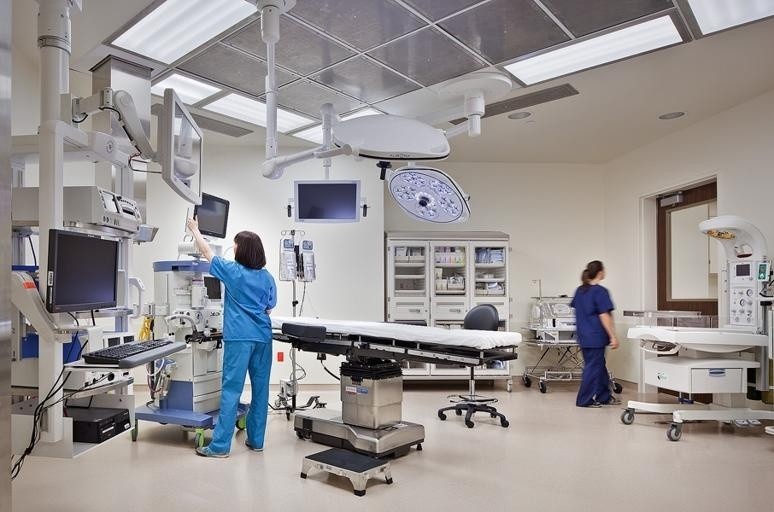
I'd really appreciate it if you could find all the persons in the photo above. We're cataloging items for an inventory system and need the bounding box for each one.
[186,214,277,459]
[569,260,622,408]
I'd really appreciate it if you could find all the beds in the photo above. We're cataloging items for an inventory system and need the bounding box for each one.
[264,313,523,457]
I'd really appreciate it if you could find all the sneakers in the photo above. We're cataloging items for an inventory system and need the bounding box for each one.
[195,446,228,458]
[607,394,621,404]
[245,438,263,451]
[587,399,602,408]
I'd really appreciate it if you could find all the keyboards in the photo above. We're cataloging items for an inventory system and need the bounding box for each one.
[81,339,174,364]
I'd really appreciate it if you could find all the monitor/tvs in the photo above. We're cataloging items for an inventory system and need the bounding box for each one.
[294,179,361,224]
[162,88,204,207]
[193,192,230,238]
[45,228,119,313]
[204,276,221,299]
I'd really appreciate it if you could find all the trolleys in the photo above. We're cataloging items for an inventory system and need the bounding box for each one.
[523,296,624,395]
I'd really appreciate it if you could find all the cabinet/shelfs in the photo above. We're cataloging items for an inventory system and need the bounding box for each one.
[385,229,511,392]
[643,355,762,406]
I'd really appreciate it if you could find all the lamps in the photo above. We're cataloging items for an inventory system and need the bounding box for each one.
[378,160,472,224]
